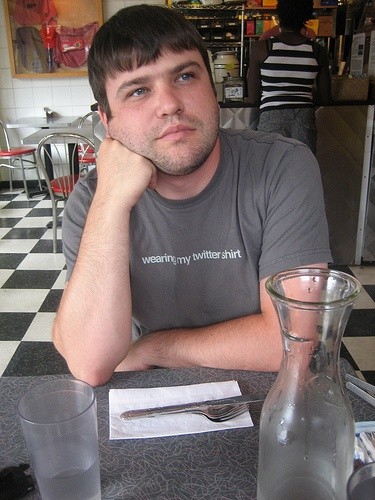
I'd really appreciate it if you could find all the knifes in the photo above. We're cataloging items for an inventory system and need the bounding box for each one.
[119,394,269,420]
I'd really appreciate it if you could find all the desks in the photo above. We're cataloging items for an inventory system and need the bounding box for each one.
[22,125,95,172]
[2,115,82,198]
[0,357,375,500]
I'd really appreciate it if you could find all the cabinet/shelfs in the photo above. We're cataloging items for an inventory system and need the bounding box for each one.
[167,6,337,75]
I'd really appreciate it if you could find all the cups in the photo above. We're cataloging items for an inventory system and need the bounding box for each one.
[346,462,374,500]
[17,378,101,500]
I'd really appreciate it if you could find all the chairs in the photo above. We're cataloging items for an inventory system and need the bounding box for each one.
[36,133,97,253]
[0,121,42,199]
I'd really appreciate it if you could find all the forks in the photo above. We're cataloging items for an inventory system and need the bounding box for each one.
[168,403,250,422]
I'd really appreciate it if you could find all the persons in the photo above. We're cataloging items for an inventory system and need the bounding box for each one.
[50,5,335,386]
[259,21,316,40]
[246,0,333,156]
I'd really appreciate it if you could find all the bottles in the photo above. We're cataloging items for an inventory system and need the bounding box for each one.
[213,50,240,83]
[257,268,362,500]
[223,76,245,103]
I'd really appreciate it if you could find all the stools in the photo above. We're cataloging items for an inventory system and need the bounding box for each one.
[78,154,96,173]
[77,144,96,156]
